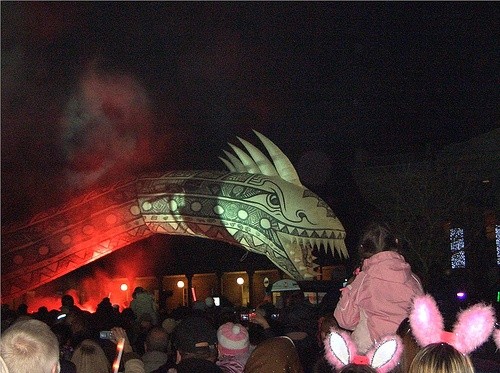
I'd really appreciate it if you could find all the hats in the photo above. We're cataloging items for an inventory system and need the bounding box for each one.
[191,301,205,310]
[172,320,212,352]
[217,323,250,356]
[132,287,144,298]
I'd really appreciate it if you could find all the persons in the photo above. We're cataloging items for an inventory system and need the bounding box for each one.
[0,286,476,373]
[334,226,425,355]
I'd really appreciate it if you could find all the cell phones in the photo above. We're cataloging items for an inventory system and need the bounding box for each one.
[100,331,116,340]
[240,314,252,321]
